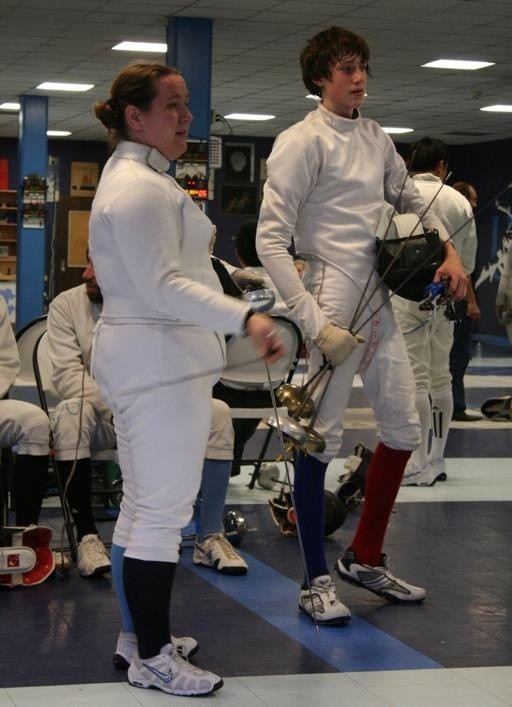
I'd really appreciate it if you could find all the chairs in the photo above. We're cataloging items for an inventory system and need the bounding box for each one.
[4,314,63,408]
[219,314,304,488]
[32,329,118,563]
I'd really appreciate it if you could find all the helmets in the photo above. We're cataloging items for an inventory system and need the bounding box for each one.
[0,523,56,590]
[373,212,447,305]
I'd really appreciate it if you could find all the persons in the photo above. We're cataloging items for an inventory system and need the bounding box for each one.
[390,138,480,486]
[0,248,250,573]
[88,64,284,696]
[254,24,469,624]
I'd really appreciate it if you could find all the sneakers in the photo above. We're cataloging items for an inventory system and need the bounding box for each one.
[192,531,249,576]
[297,574,352,627]
[335,551,427,604]
[126,644,225,697]
[76,533,112,579]
[400,460,436,490]
[428,456,448,482]
[111,629,200,668]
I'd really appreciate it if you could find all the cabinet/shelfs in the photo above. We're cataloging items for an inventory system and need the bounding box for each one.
[0,186,19,283]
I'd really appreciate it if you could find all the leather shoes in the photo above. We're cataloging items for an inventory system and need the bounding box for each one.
[452,410,482,422]
